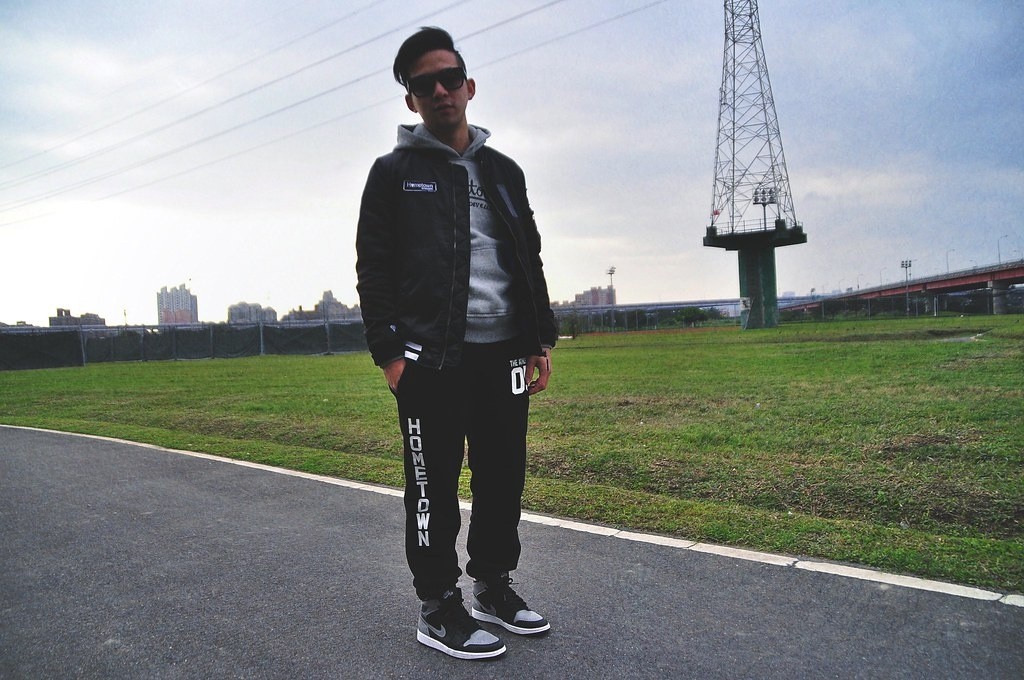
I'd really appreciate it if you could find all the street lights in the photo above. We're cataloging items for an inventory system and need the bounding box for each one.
[821,266,887,297]
[1014,250,1023,260]
[998,235,1008,264]
[970,260,978,268]
[945,248,955,273]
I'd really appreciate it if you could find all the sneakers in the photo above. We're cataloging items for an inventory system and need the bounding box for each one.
[415,584,507,659]
[471,569,550,635]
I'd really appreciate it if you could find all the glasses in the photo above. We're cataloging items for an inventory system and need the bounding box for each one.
[407,66,468,97]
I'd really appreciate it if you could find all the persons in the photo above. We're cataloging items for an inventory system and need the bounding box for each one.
[355,25,559,660]
